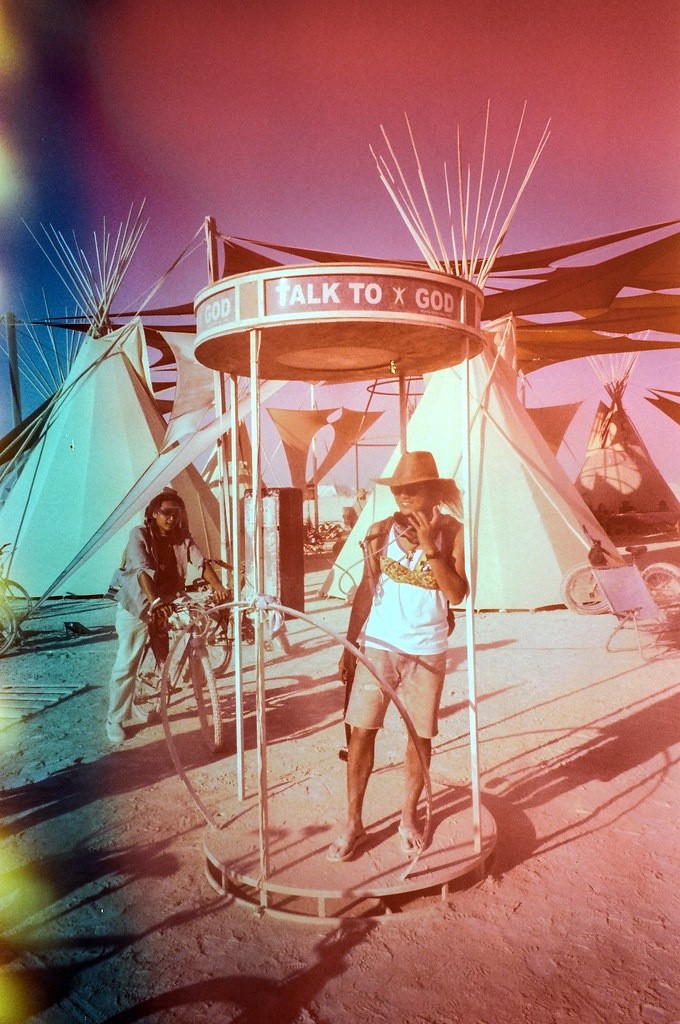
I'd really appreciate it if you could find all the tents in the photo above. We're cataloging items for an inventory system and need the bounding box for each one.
[319,348,626,611]
[0,320,221,597]
[573,400,680,512]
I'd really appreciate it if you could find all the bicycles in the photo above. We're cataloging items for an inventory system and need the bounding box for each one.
[181,593,292,682]
[0,543,32,657]
[143,588,236,750]
[565,524,680,619]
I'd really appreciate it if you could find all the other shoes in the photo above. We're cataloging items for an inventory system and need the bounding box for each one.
[104,718,125,742]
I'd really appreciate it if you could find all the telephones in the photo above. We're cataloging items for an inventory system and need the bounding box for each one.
[394,491,445,529]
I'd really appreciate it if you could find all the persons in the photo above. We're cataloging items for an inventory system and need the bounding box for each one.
[326,451,469,862]
[104,493,229,742]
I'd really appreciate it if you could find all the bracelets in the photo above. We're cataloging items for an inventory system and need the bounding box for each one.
[427,549,442,559]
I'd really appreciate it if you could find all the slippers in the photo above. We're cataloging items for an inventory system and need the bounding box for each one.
[398,825,423,854]
[324,831,368,862]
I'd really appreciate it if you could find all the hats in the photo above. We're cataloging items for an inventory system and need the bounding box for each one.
[354,488,370,499]
[368,451,464,520]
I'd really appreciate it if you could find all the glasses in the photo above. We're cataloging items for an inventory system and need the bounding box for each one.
[390,484,423,496]
[153,507,180,517]
